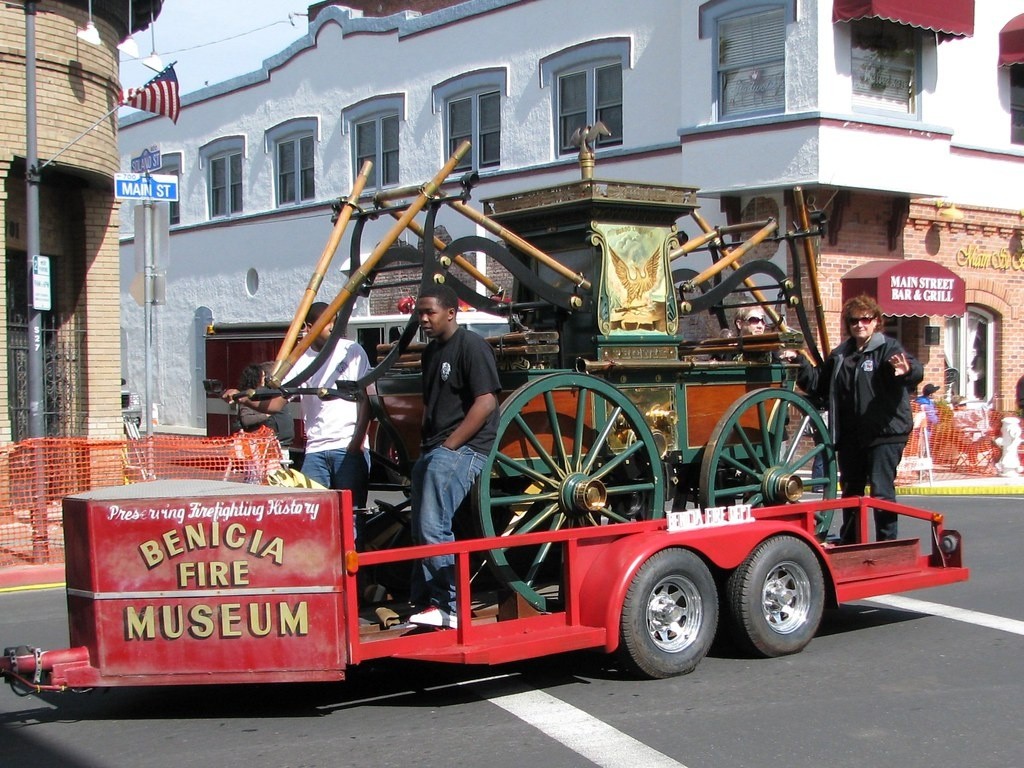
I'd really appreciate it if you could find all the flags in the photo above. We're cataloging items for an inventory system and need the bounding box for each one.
[119,64,181,126]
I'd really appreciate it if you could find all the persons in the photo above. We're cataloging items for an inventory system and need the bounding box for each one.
[705,300,924,542]
[236,363,296,469]
[220,301,378,552]
[407,285,502,626]
[914,383,968,479]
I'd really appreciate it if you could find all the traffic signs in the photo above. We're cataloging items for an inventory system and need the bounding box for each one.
[113,172,180,203]
[129,148,163,174]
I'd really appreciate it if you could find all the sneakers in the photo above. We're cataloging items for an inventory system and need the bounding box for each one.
[409,607,458,629]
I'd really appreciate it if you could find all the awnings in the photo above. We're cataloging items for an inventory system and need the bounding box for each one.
[840,260,966,320]
[833,0,1024,69]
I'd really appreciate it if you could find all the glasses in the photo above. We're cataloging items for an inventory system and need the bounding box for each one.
[847,314,877,325]
[742,316,767,325]
[959,403,967,407]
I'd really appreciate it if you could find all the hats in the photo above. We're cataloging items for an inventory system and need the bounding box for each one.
[923,384,940,396]
[951,394,969,408]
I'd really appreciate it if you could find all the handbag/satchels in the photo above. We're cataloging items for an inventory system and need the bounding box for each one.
[234,403,279,436]
